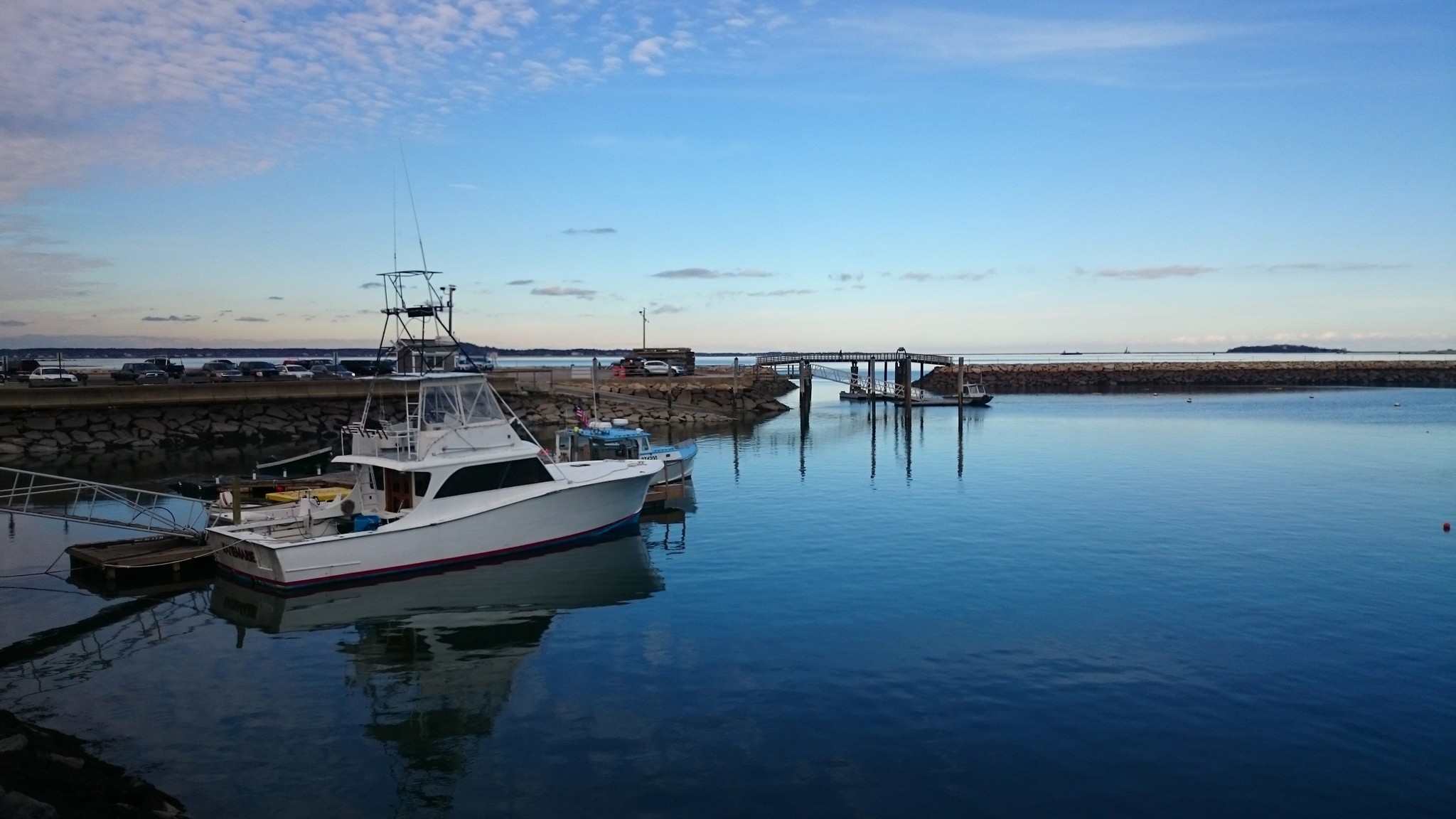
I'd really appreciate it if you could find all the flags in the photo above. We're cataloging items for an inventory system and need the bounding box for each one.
[577,407,589,427]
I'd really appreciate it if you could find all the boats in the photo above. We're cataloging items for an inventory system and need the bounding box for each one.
[199,186,664,594]
[1060,351,1084,356]
[534,405,699,485]
[941,381,994,405]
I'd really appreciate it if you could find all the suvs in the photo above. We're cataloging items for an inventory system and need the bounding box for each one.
[7,359,41,383]
[0,360,11,383]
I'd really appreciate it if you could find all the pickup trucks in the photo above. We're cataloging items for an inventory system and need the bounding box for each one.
[111,362,169,383]
[144,354,185,379]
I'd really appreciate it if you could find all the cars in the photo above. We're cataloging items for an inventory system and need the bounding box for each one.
[467,357,494,372]
[201,358,398,381]
[642,360,684,377]
[29,367,78,387]
[458,355,471,371]
